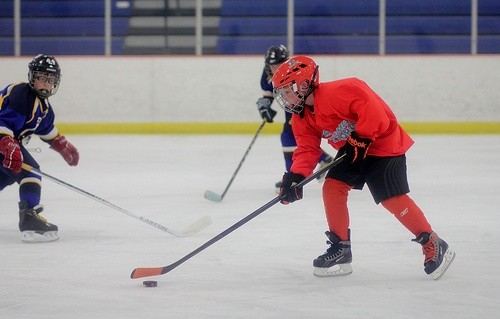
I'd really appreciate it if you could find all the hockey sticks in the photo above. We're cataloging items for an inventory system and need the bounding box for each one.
[128,152,347,280]
[202,117,266,202]
[21,163,213,239]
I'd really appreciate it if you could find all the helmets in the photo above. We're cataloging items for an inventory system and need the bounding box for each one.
[265,46,288,65]
[272,56,319,114]
[27,54,62,96]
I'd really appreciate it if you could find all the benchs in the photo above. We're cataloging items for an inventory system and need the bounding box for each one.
[0,0,500,56]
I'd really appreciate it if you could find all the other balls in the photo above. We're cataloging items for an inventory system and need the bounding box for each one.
[143,280,158,287]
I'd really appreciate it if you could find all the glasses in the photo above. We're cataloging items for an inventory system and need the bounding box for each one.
[35,76,56,84]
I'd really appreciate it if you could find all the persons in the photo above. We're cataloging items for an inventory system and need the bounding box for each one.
[271,55,455,281]
[257,47,333,191]
[0,54,80,243]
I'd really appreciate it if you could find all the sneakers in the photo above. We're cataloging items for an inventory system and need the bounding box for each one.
[316,156,333,182]
[312,229,353,276]
[18,200,58,242]
[275,179,283,194]
[411,232,455,280]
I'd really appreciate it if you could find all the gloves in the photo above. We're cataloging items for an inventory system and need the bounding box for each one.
[256,98,277,123]
[0,136,24,173]
[49,135,80,166]
[333,131,372,164]
[280,172,306,205]
